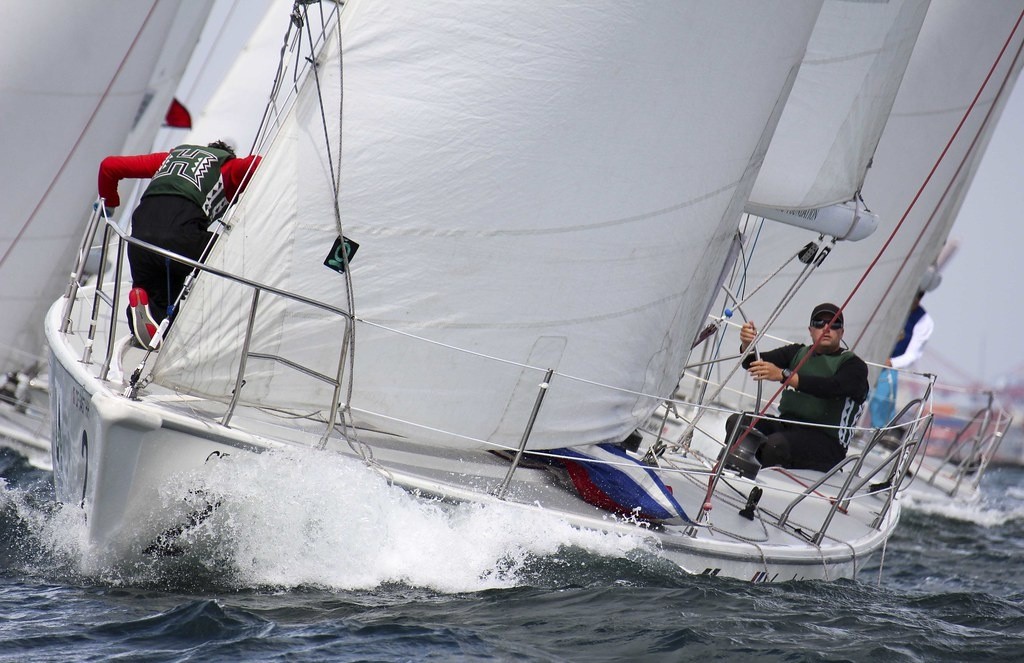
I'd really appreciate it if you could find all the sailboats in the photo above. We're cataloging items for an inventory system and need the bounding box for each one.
[0,0,1024,600]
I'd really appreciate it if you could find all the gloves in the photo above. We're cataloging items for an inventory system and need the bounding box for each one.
[92,199,115,218]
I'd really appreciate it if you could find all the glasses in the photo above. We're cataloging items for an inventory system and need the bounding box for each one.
[810,318,843,331]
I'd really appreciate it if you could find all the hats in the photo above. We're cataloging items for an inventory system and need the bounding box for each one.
[810,303,844,324]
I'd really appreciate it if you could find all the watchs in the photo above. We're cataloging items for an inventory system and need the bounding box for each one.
[781,368,791,383]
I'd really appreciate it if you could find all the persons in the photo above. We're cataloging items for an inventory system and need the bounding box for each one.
[858,288,935,438]
[726,303,869,471]
[95,138,265,354]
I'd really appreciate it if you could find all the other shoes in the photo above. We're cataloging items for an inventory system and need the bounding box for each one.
[129,288,163,352]
